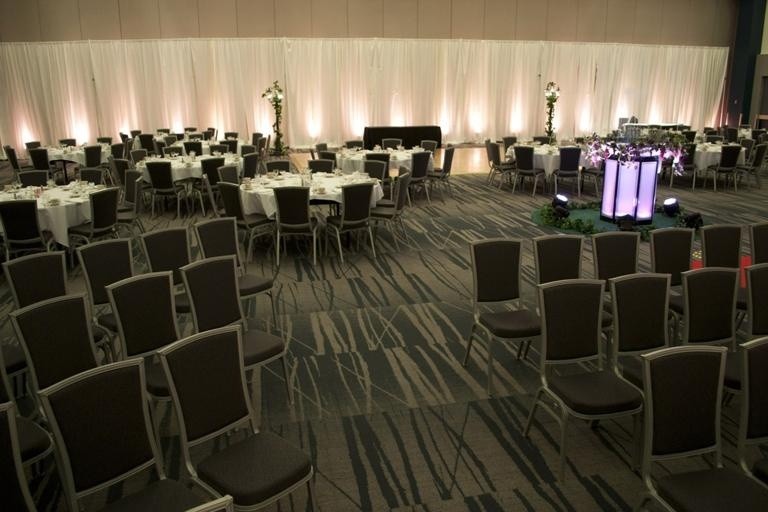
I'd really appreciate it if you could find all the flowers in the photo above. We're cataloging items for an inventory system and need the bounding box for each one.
[544,81,560,145]
[263,79,284,155]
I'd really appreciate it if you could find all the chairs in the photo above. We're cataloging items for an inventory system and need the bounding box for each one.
[484,125,766,198]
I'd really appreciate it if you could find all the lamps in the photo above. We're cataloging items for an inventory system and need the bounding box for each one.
[552,194,569,210]
[664,197,680,218]
